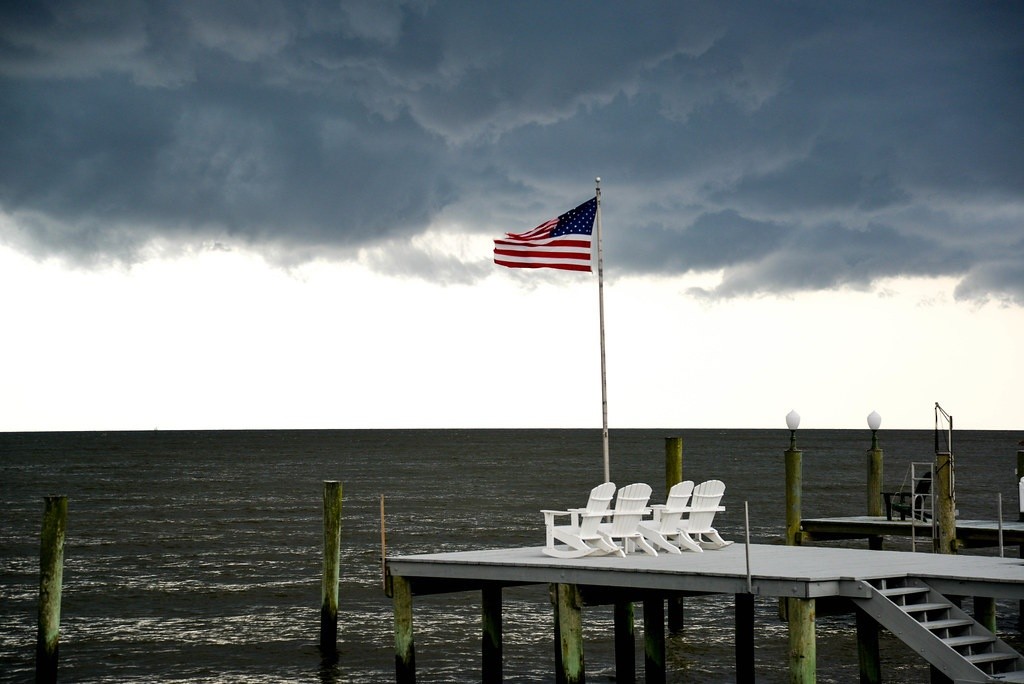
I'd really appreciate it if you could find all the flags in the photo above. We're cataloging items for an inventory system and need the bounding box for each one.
[493,197,598,273]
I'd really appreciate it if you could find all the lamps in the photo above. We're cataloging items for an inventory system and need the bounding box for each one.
[867,410,882,452]
[786,410,800,453]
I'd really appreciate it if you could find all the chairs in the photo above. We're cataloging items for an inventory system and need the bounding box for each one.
[637,480,704,555]
[540,483,624,558]
[569,483,658,558]
[880,471,932,523]
[651,480,734,549]
